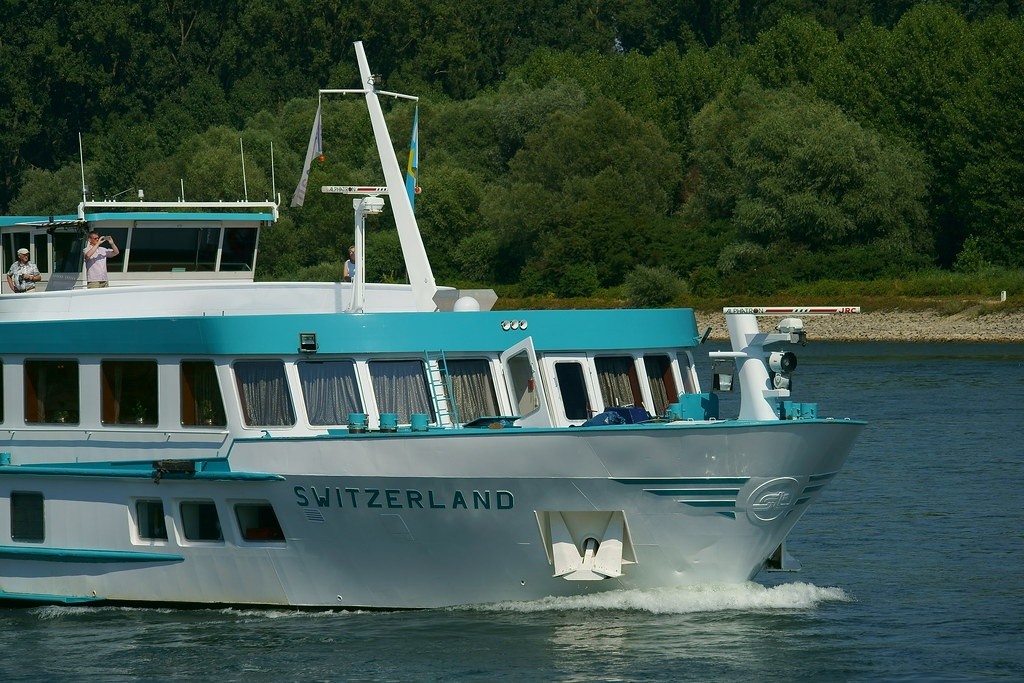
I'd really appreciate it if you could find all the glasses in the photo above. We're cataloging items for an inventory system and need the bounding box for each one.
[90,237,99,241]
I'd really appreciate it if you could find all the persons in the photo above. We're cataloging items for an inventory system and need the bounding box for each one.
[343,246,355,282]
[83,231,119,288]
[7,249,41,293]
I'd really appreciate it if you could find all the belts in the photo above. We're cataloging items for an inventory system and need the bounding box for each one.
[15,286,35,293]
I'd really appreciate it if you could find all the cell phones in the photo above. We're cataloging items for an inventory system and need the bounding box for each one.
[104,236,110,241]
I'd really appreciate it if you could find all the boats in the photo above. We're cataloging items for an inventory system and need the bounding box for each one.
[0,41,868,611]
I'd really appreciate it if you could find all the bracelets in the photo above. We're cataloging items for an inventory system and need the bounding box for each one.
[31,276,34,281]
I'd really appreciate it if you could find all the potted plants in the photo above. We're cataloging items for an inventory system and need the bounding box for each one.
[131,399,148,424]
[199,399,217,426]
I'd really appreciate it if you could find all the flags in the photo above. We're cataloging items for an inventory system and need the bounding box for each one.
[406,110,418,210]
[290,107,322,207]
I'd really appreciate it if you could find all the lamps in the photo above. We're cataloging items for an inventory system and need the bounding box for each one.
[299,334,318,351]
[697,327,714,345]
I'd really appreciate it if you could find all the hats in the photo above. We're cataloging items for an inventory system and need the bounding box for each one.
[17,248,30,255]
